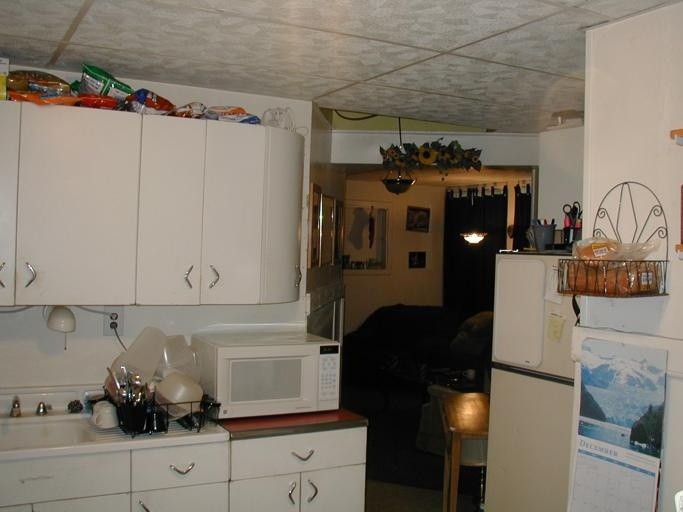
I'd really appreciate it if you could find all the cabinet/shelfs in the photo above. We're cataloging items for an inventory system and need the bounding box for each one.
[0,441,231,512]
[229,426,368,512]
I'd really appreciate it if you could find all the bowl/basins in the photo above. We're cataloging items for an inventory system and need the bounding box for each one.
[104,326,204,418]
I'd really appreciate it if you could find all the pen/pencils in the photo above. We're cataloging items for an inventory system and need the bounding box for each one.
[576,219,582,229]
[537,218,555,225]
[564,215,571,228]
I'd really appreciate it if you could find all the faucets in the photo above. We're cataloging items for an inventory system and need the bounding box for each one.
[10,403,21,417]
[37,402,47,416]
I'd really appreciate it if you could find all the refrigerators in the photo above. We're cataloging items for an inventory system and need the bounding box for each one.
[481,243,584,511]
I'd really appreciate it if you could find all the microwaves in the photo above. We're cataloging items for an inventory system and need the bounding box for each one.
[192,331,343,422]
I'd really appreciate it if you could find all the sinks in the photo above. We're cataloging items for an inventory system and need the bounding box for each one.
[0,418,84,452]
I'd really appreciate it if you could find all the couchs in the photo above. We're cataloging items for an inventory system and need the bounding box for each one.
[340,304,493,497]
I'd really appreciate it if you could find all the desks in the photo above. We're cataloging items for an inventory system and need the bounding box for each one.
[438,391,490,512]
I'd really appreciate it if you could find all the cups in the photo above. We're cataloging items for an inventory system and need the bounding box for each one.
[125,400,147,432]
[145,411,169,432]
[530,223,557,252]
[92,400,120,433]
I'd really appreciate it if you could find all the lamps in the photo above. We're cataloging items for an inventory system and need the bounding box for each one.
[380,117,415,195]
[46,307,76,350]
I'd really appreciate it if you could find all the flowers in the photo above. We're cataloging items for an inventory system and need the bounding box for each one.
[379,136,483,171]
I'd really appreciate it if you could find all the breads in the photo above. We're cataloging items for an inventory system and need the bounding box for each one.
[567,241,659,296]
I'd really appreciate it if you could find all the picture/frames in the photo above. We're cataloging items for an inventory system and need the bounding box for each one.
[405,205,431,233]
[318,193,336,267]
[342,199,393,276]
[407,249,427,271]
[307,182,323,269]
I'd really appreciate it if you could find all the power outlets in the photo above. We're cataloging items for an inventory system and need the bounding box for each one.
[103,306,124,337]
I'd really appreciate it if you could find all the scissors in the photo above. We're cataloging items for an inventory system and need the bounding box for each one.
[562,202,580,227]
